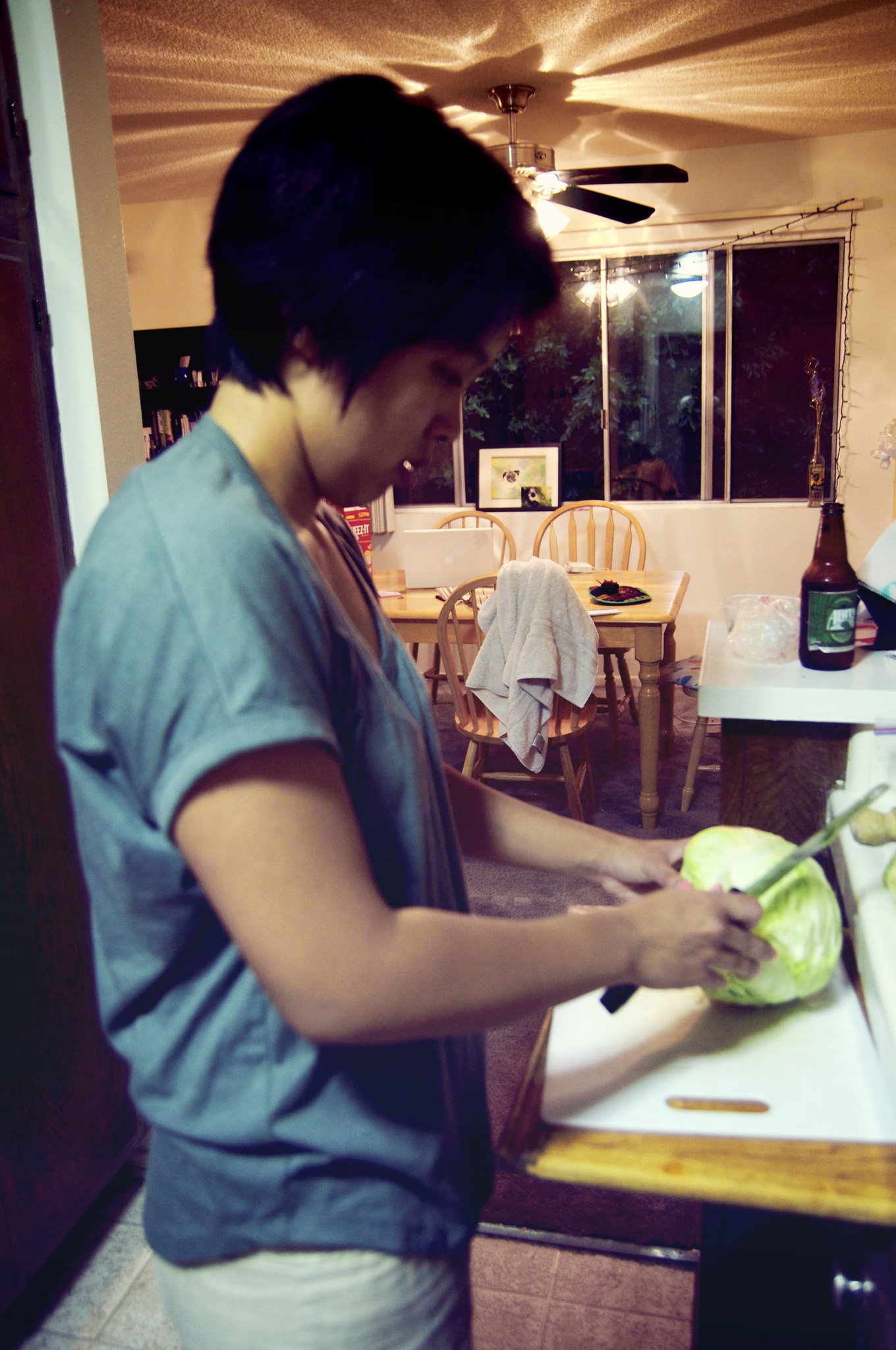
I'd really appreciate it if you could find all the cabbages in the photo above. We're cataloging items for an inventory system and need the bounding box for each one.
[678,813,843,1008]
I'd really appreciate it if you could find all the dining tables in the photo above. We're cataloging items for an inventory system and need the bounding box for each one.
[368,567,694,831]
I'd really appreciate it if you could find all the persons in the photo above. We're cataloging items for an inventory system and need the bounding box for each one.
[52,64,781,1350]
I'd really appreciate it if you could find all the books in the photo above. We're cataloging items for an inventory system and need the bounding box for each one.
[142,405,208,465]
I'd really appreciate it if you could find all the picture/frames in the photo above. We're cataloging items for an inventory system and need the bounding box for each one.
[473,441,565,513]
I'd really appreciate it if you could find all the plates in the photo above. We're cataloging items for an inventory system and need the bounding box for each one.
[588,587,651,604]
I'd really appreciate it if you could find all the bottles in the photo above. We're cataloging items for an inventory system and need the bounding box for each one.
[799,501,858,671]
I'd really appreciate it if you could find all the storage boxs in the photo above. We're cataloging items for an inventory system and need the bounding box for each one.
[338,505,374,589]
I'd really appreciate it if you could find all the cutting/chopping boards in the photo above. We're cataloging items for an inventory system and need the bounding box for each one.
[539,913,896,1145]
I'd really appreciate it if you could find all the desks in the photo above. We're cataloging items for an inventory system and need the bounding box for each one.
[698,605,896,934]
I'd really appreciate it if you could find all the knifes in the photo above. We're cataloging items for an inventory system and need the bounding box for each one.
[600,782,888,1013]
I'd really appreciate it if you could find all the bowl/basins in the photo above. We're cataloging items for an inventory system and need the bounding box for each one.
[719,595,801,666]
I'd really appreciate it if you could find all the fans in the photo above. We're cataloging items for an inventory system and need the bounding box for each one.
[479,81,692,229]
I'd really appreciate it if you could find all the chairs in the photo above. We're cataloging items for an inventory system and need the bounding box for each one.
[426,570,603,825]
[396,508,518,729]
[524,500,649,753]
[653,649,724,816]
